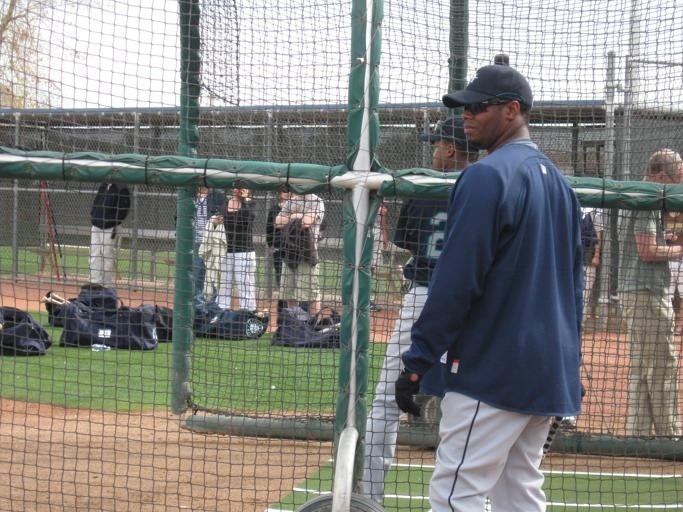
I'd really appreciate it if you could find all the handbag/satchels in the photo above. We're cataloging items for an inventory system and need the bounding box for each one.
[0,306,52,357]
[194,301,269,341]
[45,283,173,351]
[271,303,340,348]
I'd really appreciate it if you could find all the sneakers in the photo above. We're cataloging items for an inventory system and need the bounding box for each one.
[560,416,577,430]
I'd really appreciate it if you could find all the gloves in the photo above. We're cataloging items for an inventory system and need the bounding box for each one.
[393,369,422,418]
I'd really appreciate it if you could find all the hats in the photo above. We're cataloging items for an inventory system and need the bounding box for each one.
[419,112,467,147]
[441,64,533,110]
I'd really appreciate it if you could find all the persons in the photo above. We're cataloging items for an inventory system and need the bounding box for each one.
[393,60,584,511]
[577,205,601,334]
[207,186,257,316]
[172,183,229,318]
[356,113,478,509]
[659,208,682,337]
[272,191,325,326]
[263,188,291,327]
[369,205,389,312]
[611,147,682,442]
[87,182,131,289]
